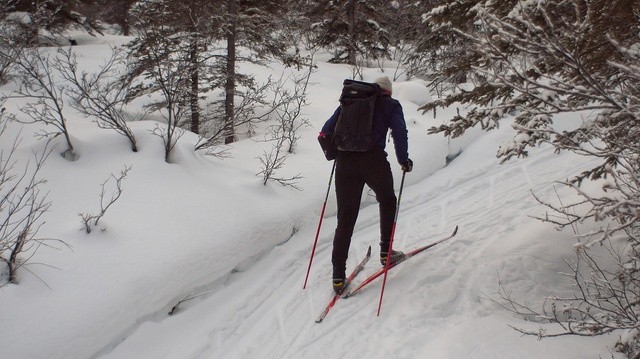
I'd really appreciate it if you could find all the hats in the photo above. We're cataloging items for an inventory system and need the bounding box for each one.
[377,76,392,94]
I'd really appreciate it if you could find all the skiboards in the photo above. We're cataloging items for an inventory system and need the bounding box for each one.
[314,225,460,323]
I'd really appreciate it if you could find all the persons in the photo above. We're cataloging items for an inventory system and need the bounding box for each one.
[317,76,414,295]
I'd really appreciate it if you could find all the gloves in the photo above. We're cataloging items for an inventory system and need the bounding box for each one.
[398,154,413,171]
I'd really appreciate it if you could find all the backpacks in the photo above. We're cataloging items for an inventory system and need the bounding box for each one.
[333,78,380,151]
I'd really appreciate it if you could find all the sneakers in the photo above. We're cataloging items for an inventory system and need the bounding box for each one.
[333,278,349,293]
[380,246,403,265]
